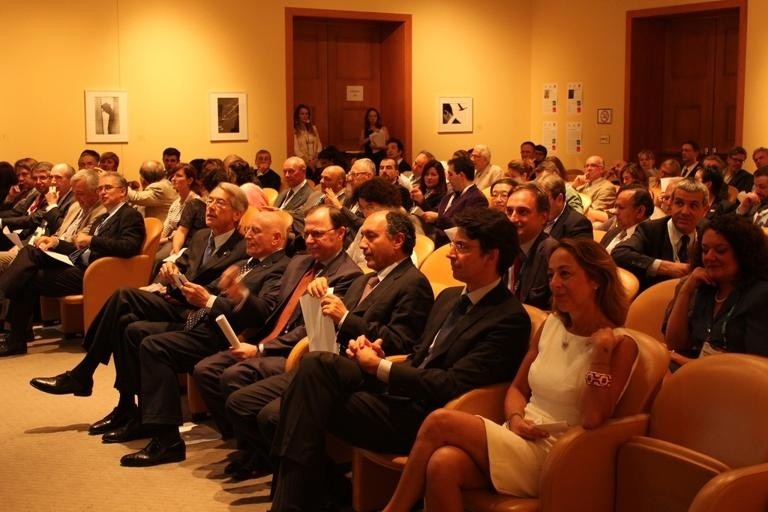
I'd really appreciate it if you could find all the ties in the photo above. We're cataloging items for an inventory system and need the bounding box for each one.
[511,254,521,290]
[431,296,469,351]
[451,192,461,206]
[69,214,109,265]
[188,259,257,329]
[31,194,44,211]
[261,262,322,344]
[358,276,379,306]
[678,235,690,262]
[203,237,215,265]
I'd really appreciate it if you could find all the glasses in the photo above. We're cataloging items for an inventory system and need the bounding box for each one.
[450,240,479,251]
[304,228,337,237]
[97,186,122,192]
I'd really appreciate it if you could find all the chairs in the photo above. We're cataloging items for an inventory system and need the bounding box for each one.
[538,353,768,512]
[413,233,435,270]
[261,187,278,208]
[443,328,671,512]
[351,304,548,512]
[625,278,682,342]
[286,285,450,373]
[419,240,464,287]
[58,218,164,333]
[617,266,640,315]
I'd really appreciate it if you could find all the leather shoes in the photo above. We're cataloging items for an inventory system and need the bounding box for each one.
[89,407,137,434]
[120,440,186,466]
[30,371,93,396]
[102,419,152,442]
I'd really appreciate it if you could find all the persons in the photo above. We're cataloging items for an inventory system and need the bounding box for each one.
[293,104,322,171]
[0,140,767,511]
[360,107,390,175]
[443,104,462,124]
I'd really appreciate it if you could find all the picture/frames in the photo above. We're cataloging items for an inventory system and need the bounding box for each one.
[436,97,473,132]
[85,90,129,143]
[209,92,248,142]
